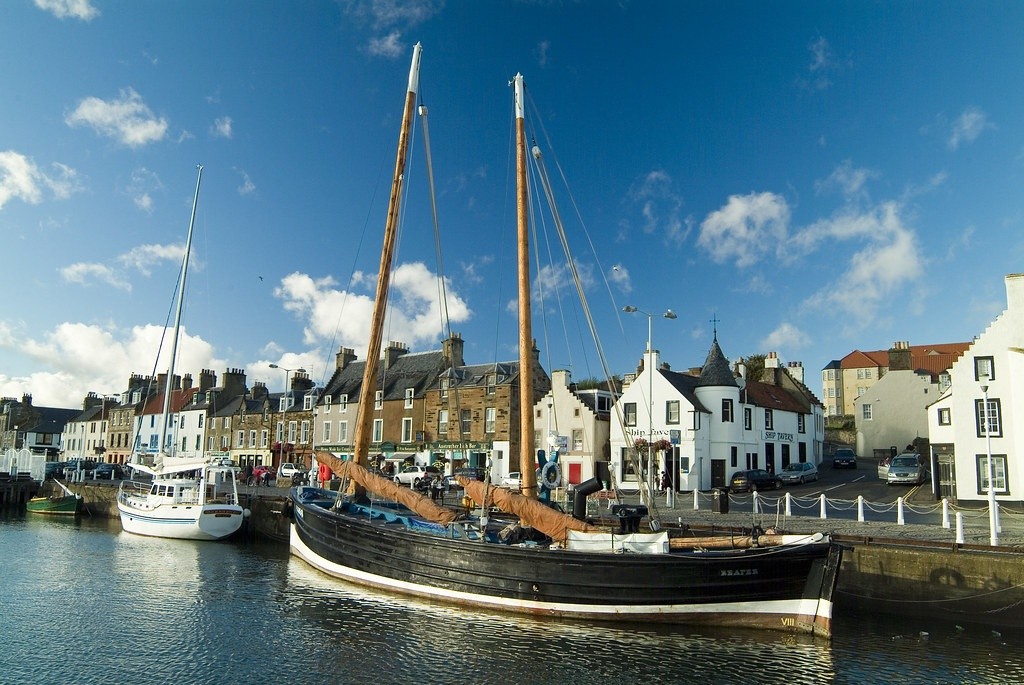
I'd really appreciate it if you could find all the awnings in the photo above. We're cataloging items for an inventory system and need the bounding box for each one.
[385,452,414,462]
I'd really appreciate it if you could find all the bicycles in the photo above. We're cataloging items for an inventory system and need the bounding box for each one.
[246,473,266,487]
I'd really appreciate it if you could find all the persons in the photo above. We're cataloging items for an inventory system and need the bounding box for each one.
[880,456,892,467]
[388,464,392,473]
[767,464,774,474]
[417,470,447,500]
[654,473,661,490]
[262,471,272,487]
[244,461,254,487]
[662,470,672,489]
[403,461,413,468]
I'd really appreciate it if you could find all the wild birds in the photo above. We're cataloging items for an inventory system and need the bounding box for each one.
[259,277,263,281]
[613,266,618,272]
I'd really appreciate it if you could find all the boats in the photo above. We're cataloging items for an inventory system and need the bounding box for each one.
[25,476,84,517]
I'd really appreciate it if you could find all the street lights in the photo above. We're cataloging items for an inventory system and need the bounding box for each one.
[979,373,999,547]
[623,305,679,495]
[268,363,309,477]
[89,391,121,463]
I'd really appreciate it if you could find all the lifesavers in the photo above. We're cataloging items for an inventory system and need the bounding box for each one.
[542,461,562,489]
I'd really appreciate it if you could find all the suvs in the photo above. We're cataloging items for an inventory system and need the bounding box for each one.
[64,460,97,479]
[443,467,491,491]
[878,453,927,485]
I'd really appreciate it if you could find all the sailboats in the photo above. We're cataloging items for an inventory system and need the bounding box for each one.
[115,155,249,542]
[283,42,866,643]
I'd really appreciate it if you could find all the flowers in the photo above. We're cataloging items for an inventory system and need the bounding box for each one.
[633,438,672,455]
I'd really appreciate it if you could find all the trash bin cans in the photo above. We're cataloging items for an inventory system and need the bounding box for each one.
[712,486,728,512]
[890,446,897,458]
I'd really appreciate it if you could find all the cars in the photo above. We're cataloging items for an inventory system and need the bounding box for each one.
[309,464,396,480]
[252,465,277,480]
[89,463,124,480]
[44,461,68,479]
[393,465,442,488]
[730,468,783,494]
[832,447,857,468]
[278,462,311,477]
[777,461,820,486]
[502,471,523,492]
